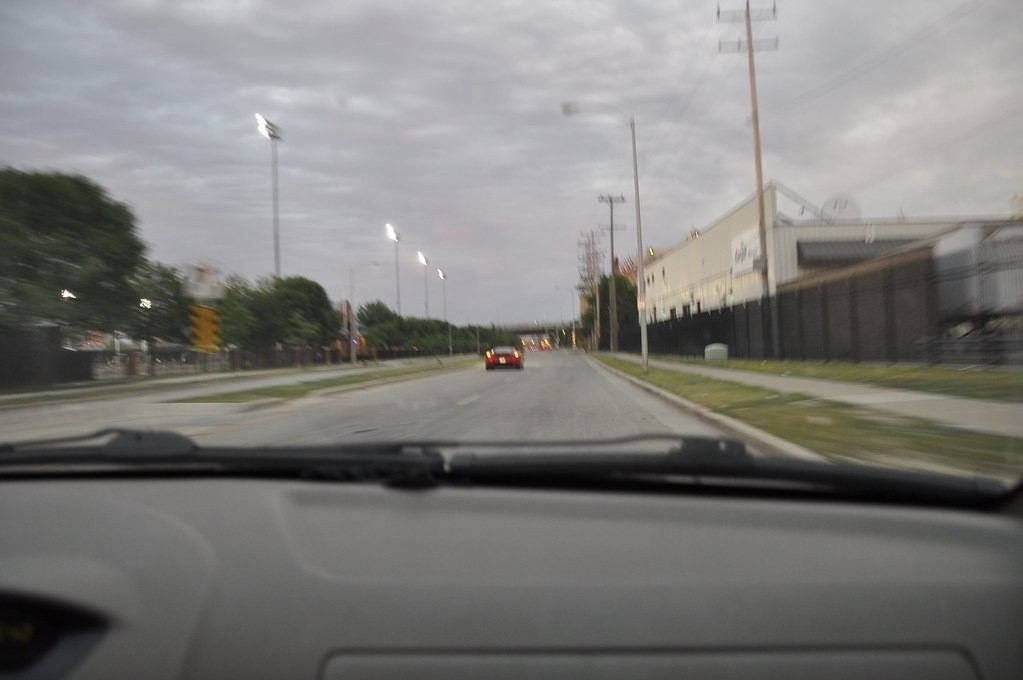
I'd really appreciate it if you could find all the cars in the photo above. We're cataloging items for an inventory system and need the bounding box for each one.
[485,344,524,371]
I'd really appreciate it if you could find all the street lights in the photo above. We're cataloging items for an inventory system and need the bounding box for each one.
[437,268,453,357]
[254,110,283,280]
[563,102,651,376]
[417,249,430,320]
[386,223,403,361]
